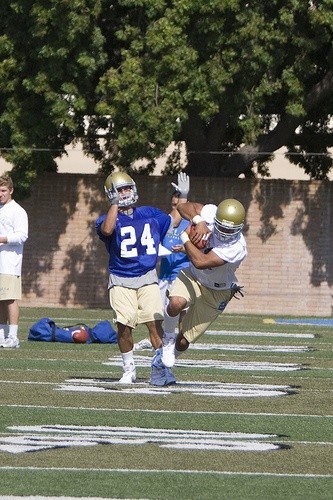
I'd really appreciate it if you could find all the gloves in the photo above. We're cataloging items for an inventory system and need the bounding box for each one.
[171,172,190,199]
[104,182,120,205]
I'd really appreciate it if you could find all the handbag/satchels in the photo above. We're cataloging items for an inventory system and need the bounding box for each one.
[28,317,119,344]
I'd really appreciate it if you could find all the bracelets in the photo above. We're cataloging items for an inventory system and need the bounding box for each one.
[180,232,190,244]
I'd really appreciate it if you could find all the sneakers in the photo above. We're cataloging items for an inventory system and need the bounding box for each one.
[133,337,153,351]
[1,336,19,348]
[161,334,176,367]
[0,336,5,347]
[151,354,164,369]
[119,366,136,382]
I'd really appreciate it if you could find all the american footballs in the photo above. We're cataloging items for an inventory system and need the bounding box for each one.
[190,225,206,249]
[72,327,89,343]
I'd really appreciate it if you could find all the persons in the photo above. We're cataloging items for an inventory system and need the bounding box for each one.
[0,171,28,348]
[149,198,247,370]
[96,172,190,386]
[139,192,193,384]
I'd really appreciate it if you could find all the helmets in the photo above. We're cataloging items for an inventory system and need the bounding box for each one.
[104,172,138,207]
[213,199,246,242]
